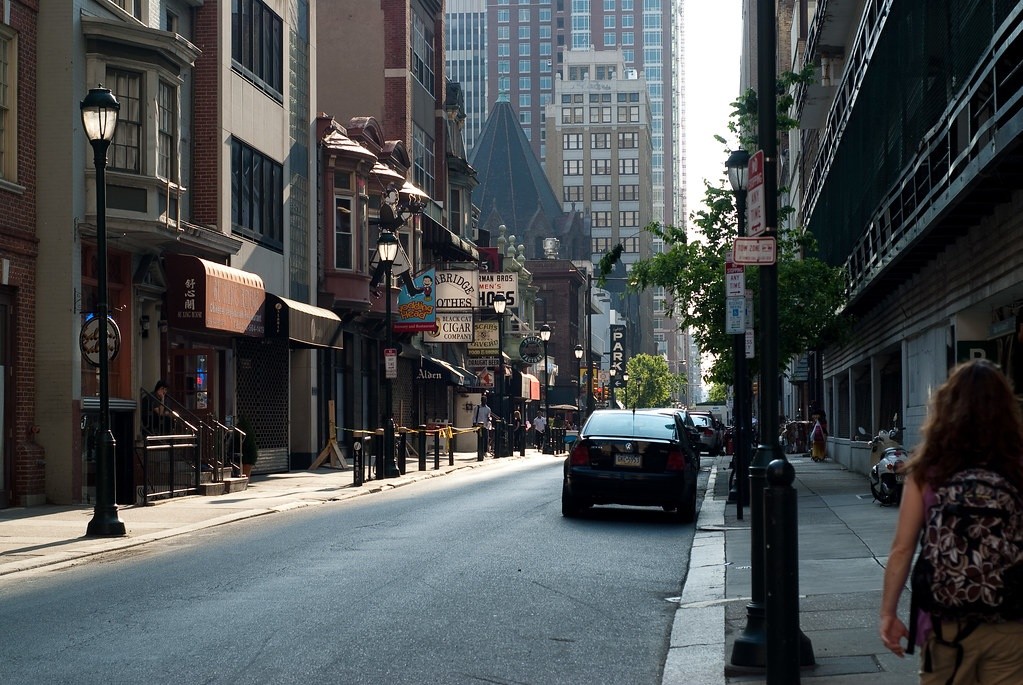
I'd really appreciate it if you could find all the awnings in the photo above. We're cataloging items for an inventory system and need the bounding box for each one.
[511,368,540,403]
[421,354,481,388]
[164,252,265,342]
[265,287,344,351]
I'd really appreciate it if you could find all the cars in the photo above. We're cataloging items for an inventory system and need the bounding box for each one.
[560,409,700,524]
[641,407,730,474]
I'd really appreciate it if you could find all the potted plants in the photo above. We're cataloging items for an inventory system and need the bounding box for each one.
[228,417,259,477]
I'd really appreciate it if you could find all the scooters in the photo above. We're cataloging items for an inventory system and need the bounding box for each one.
[857,412,910,506]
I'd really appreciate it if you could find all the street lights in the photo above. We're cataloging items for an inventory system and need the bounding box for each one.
[637,377,642,408]
[539,324,552,456]
[723,148,753,505]
[573,344,584,434]
[492,291,507,459]
[622,372,629,410]
[376,228,402,480]
[78,82,127,540]
[609,366,617,409]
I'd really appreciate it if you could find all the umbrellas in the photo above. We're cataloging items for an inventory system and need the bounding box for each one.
[540,404,579,426]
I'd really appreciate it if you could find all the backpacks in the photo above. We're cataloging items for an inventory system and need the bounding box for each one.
[913,464,1023,622]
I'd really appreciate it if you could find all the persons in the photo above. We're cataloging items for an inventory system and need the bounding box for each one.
[715,418,726,456]
[512,411,526,456]
[880,359,1023,685]
[807,399,828,451]
[472,395,492,461]
[532,410,579,451]
[140,380,180,434]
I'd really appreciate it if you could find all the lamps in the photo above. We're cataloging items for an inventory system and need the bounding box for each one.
[139,315,150,329]
[157,320,168,332]
